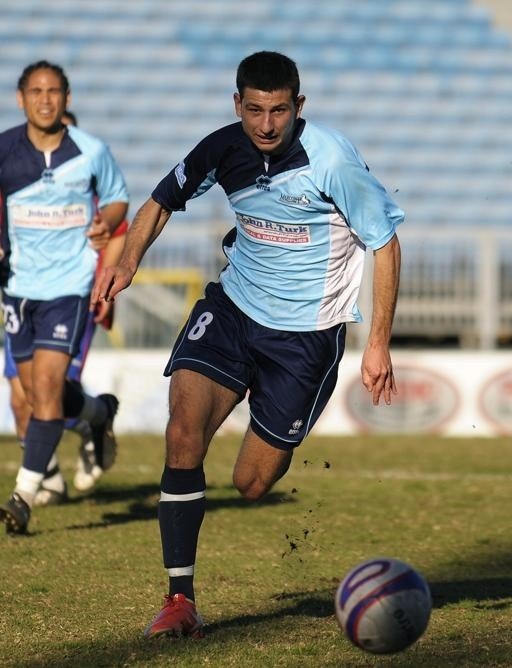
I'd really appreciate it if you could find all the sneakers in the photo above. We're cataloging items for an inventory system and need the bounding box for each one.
[0,493,29,536]
[73,394,117,490]
[144,593,205,639]
[33,482,67,508]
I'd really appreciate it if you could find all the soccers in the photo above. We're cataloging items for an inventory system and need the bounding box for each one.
[335,555,431,656]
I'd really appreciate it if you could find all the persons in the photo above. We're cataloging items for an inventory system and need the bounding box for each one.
[97,51,406,640]
[0,59,119,537]
[4,111,128,508]
